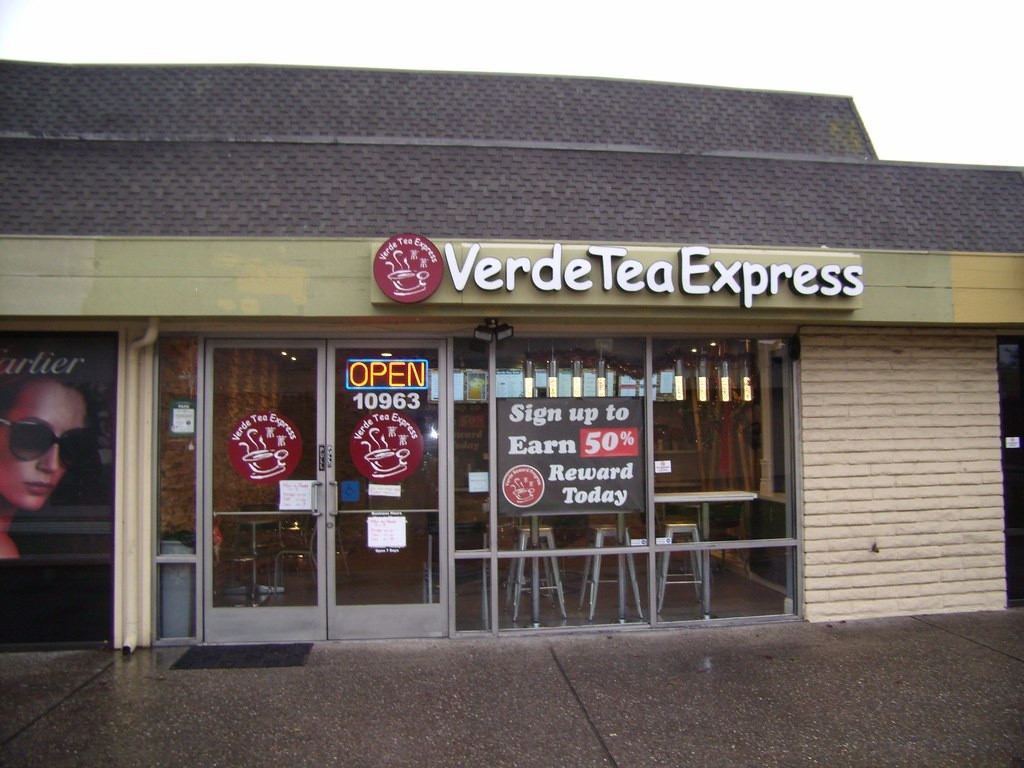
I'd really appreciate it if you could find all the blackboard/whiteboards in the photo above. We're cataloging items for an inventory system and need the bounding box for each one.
[495,397,645,515]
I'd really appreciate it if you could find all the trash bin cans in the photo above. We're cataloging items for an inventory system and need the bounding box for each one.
[159,537,195,637]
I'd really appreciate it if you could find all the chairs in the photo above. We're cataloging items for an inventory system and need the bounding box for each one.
[230,503,352,606]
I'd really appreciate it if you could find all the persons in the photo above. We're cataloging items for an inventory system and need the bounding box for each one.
[0,373,98,558]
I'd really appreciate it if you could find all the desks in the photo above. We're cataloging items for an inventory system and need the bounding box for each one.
[471,490,757,629]
[223,515,290,606]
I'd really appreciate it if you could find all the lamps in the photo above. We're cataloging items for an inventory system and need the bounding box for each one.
[472,318,513,341]
[696,340,710,402]
[595,338,608,398]
[521,336,536,398]
[545,338,559,398]
[571,360,585,398]
[740,339,753,401]
[717,340,732,402]
[672,334,687,401]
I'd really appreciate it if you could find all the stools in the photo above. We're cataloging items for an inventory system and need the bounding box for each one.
[504,525,569,622]
[657,520,703,613]
[424,513,491,631]
[578,524,645,621]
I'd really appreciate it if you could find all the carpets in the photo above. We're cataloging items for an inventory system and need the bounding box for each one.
[169,642,315,670]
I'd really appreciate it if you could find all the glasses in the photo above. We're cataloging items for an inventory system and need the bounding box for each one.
[0,418,100,475]
